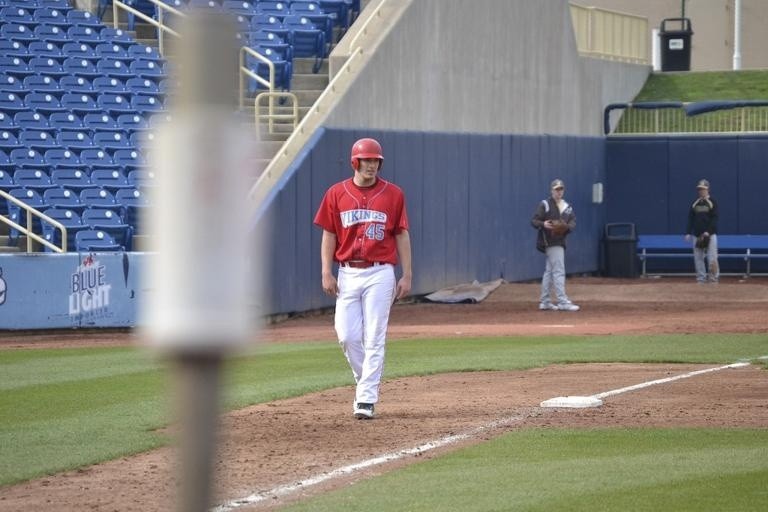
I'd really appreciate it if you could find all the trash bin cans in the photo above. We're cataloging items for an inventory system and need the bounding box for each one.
[658,17,694,72]
[602,222,640,278]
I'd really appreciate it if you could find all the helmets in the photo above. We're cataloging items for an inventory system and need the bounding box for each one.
[350,138,383,170]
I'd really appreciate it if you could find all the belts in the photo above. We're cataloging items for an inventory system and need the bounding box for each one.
[341,259,384,268]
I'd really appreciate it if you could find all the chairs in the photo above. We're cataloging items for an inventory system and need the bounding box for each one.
[0,0,359,254]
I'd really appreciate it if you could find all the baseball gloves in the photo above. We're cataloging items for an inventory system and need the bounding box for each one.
[552,218,569,235]
[696,234,709,248]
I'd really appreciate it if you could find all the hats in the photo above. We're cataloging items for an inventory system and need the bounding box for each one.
[551,178,565,190]
[696,179,710,189]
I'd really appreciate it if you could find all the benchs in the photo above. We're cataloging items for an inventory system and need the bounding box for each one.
[635,234,768,280]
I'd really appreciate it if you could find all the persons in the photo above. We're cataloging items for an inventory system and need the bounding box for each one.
[313,138,412,419]
[531,179,580,311]
[684,178,719,285]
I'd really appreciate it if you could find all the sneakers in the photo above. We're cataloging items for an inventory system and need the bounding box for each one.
[353,403,375,418]
[539,300,581,312]
